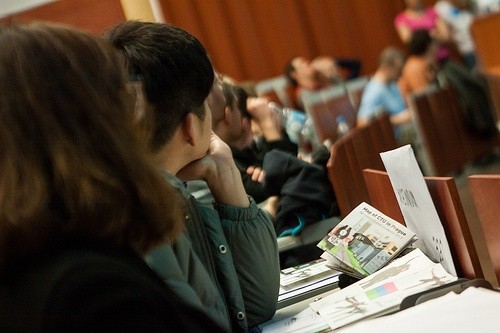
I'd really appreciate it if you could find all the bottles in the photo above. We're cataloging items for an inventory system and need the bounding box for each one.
[335,115,352,138]
[268,102,313,137]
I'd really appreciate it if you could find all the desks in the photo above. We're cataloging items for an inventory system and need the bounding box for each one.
[254,257,500,333]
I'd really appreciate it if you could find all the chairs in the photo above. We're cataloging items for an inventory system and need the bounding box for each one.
[260,6,500,290]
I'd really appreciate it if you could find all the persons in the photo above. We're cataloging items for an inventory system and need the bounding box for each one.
[0,21,229,333]
[176,1,480,228]
[105,17,277,329]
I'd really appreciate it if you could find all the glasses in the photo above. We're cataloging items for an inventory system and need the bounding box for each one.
[120,75,146,126]
